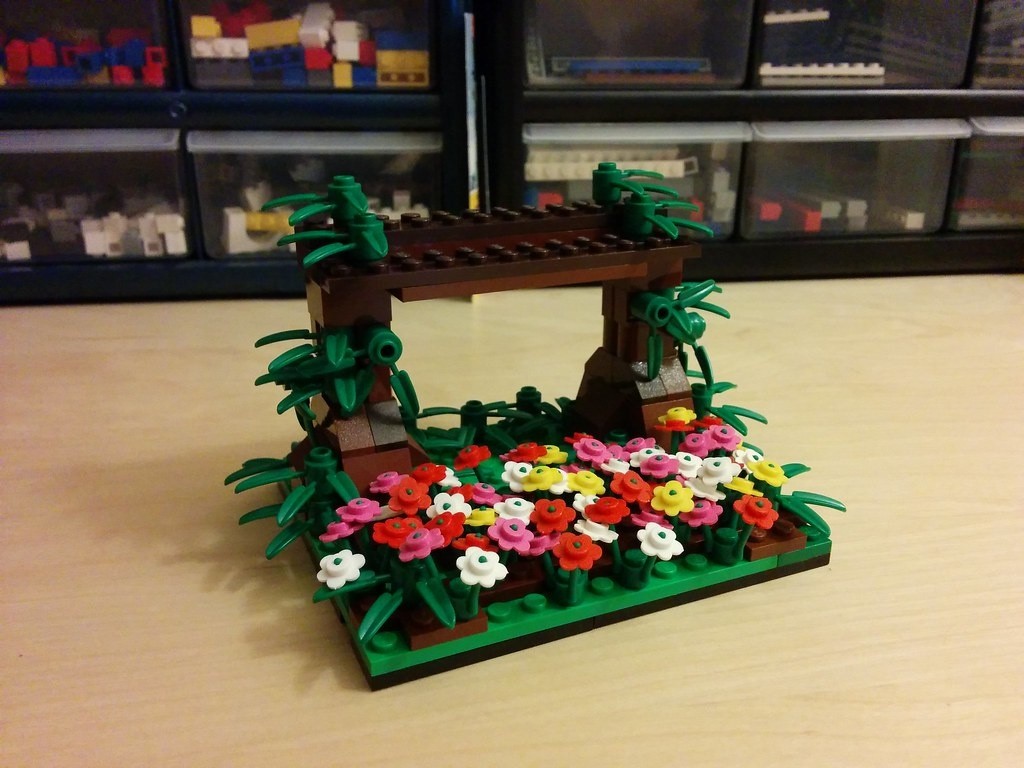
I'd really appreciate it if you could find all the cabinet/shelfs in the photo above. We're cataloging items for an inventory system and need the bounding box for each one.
[0,0,497,309]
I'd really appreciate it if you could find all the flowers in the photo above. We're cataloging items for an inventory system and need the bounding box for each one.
[319,408,783,590]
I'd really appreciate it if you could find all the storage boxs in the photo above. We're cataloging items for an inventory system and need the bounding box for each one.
[186,134,444,260]
[738,119,973,237]
[523,123,752,236]
[177,3,433,92]
[972,0,1024,89]
[948,117,1024,234]
[0,0,170,88]
[0,132,185,264]
[753,0,979,92]
